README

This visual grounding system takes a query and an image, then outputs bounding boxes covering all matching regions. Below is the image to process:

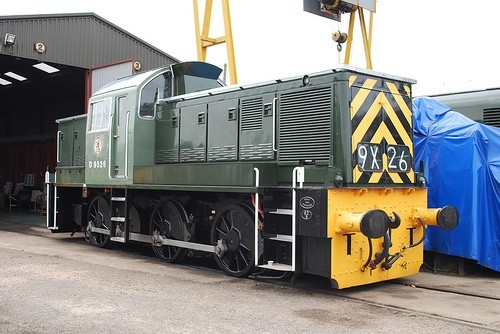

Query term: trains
[46,0,459,290]
